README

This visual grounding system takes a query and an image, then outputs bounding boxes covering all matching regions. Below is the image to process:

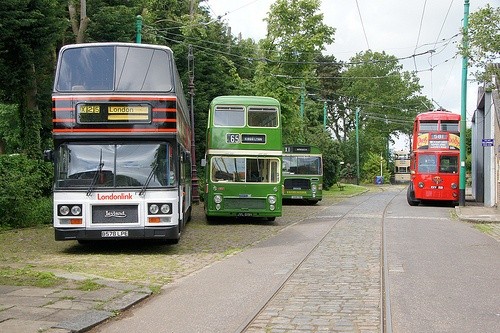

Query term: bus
[44,42,192,244]
[407,98,461,206]
[201,95,282,221]
[282,144,323,204]
